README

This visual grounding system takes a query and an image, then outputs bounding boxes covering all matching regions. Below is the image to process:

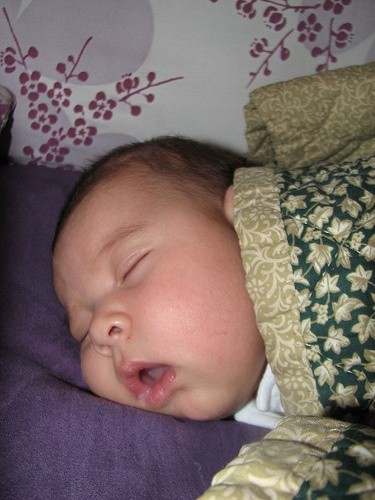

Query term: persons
[50,136,375,431]
